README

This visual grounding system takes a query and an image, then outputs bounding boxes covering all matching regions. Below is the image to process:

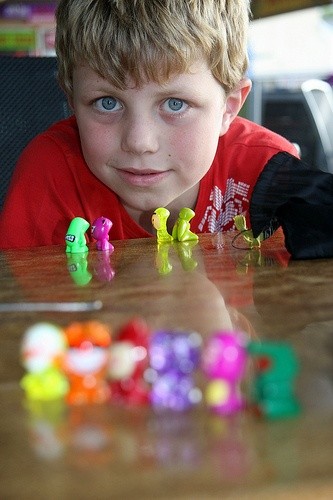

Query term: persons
[1,0,297,255]
